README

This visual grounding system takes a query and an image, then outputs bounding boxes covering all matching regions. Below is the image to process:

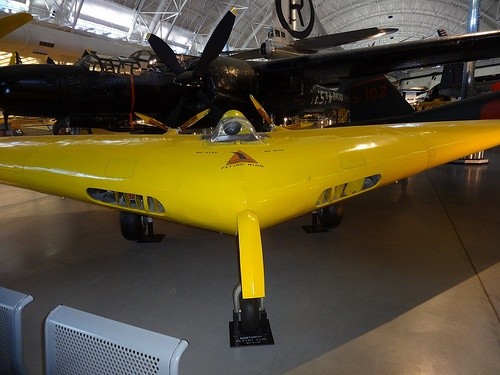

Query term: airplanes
[1,6,500,138]
[0,93,500,333]
[213,26,399,61]
